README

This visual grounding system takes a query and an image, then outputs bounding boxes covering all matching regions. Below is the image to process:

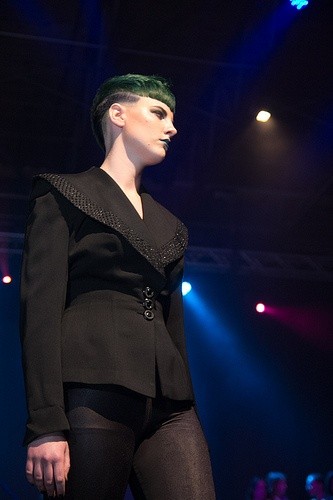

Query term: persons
[20,73,219,499]
[247,471,333,500]
